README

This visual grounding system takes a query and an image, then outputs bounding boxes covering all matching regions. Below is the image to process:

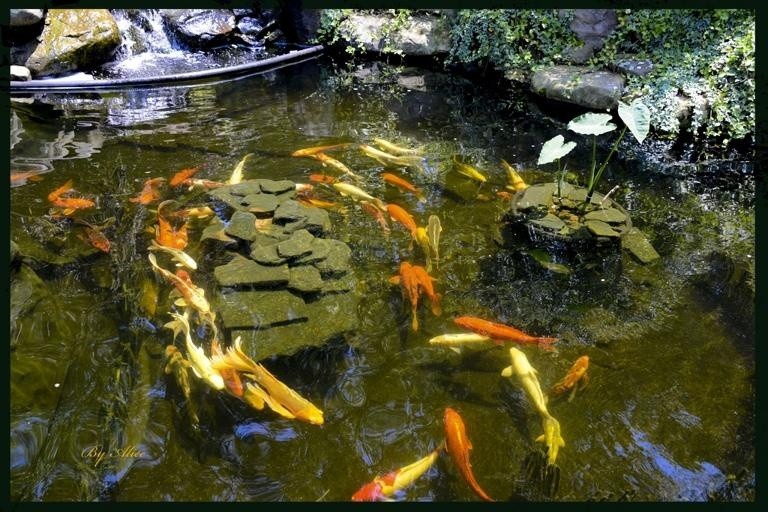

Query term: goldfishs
[292,137,530,331]
[10,153,324,426]
